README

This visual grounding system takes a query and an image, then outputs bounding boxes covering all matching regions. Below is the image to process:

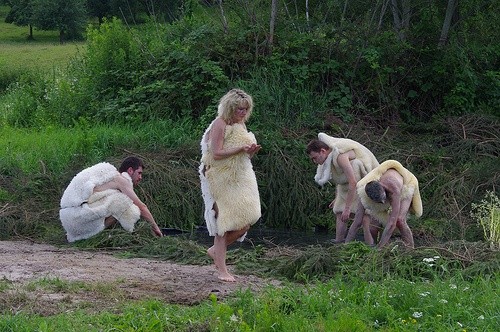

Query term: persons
[356,160,424,253]
[304,132,380,245]
[199,88,262,282]
[59,157,164,244]
